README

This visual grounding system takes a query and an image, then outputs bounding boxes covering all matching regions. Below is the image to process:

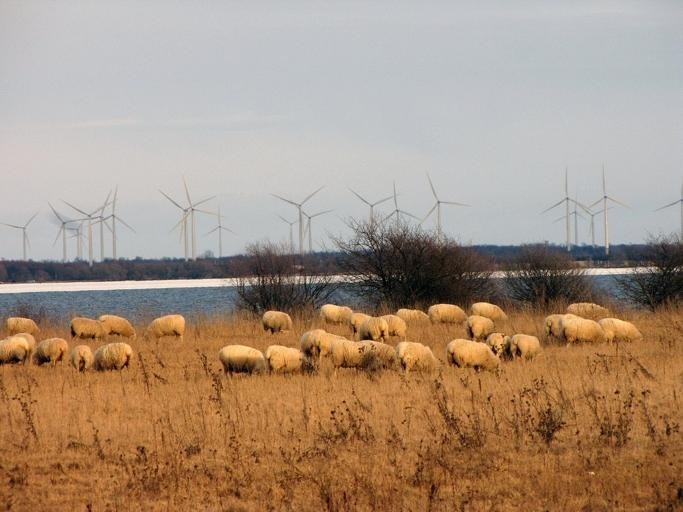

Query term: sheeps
[543,300,644,345]
[319,300,513,334]
[445,333,542,375]
[143,314,186,342]
[259,310,294,334]
[0,314,136,373]
[216,329,439,376]
